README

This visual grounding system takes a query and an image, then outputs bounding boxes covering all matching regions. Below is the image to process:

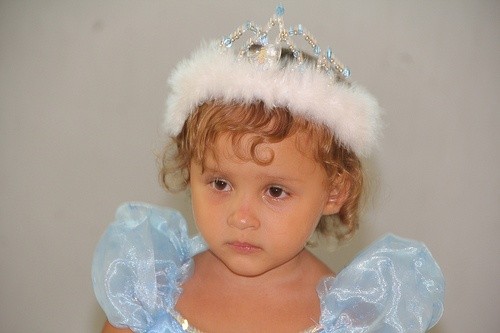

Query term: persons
[88,2,447,332]
